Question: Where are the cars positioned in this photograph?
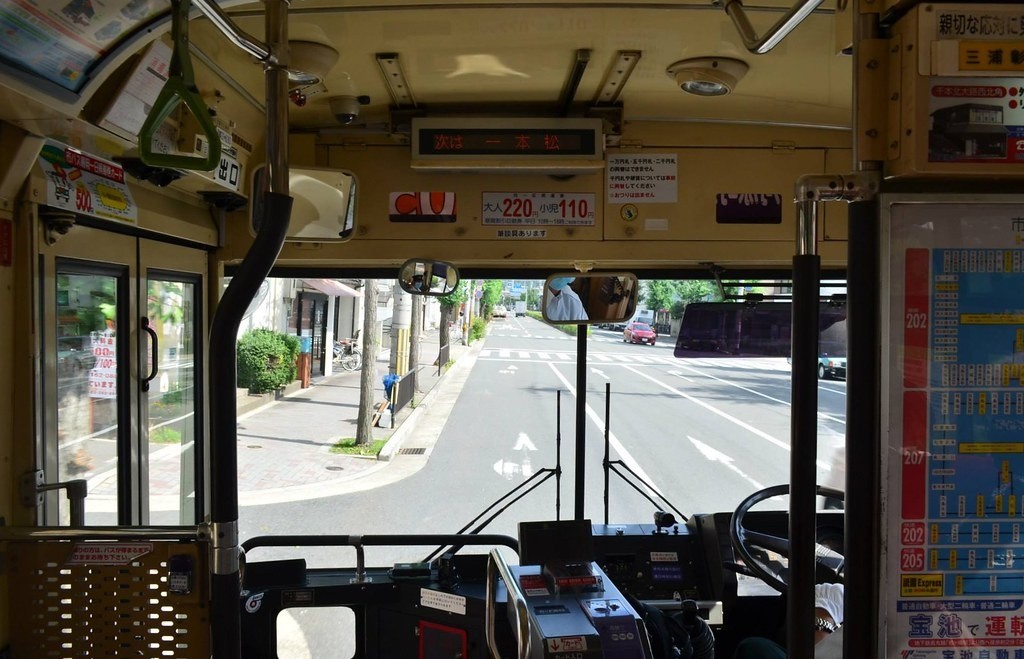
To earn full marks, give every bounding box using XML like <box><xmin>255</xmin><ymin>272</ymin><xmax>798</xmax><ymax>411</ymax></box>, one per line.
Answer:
<box><xmin>56</xmin><ymin>335</ymin><xmax>96</xmax><ymax>377</ymax></box>
<box><xmin>623</xmin><ymin>322</ymin><xmax>656</xmax><ymax>345</ymax></box>
<box><xmin>492</xmin><ymin>306</ymin><xmax>507</xmax><ymax>317</ymax></box>
<box><xmin>787</xmin><ymin>356</ymin><xmax>846</xmax><ymax>379</ymax></box>
<box><xmin>593</xmin><ymin>321</ymin><xmax>627</xmax><ymax>332</ymax></box>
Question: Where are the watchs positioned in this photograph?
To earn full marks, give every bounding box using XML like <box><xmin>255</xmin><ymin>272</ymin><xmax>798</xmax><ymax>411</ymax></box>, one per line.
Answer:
<box><xmin>814</xmin><ymin>617</ymin><xmax>834</xmax><ymax>633</ymax></box>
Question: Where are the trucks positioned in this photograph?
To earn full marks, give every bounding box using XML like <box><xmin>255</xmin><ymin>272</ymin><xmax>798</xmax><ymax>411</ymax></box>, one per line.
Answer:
<box><xmin>516</xmin><ymin>300</ymin><xmax>527</xmax><ymax>318</ymax></box>
<box><xmin>626</xmin><ymin>304</ymin><xmax>655</xmax><ymax>333</ymax></box>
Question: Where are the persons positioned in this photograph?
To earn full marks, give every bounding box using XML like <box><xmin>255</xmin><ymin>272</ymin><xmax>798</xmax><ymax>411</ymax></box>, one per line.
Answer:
<box><xmin>409</xmin><ymin>275</ymin><xmax>423</xmax><ymax>292</ymax></box>
<box><xmin>738</xmin><ymin>578</ymin><xmax>845</xmax><ymax>659</ymax></box>
<box><xmin>545</xmin><ymin>277</ymin><xmax>590</xmax><ymax>320</ymax></box>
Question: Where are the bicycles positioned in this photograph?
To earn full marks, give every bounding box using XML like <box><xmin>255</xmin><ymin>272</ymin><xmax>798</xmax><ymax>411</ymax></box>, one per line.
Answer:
<box><xmin>333</xmin><ymin>338</ymin><xmax>363</xmax><ymax>372</ymax></box>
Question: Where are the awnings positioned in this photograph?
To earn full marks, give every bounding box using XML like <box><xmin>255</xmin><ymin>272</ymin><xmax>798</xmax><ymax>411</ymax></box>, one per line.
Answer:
<box><xmin>300</xmin><ymin>278</ymin><xmax>362</xmax><ymax>298</ymax></box>
